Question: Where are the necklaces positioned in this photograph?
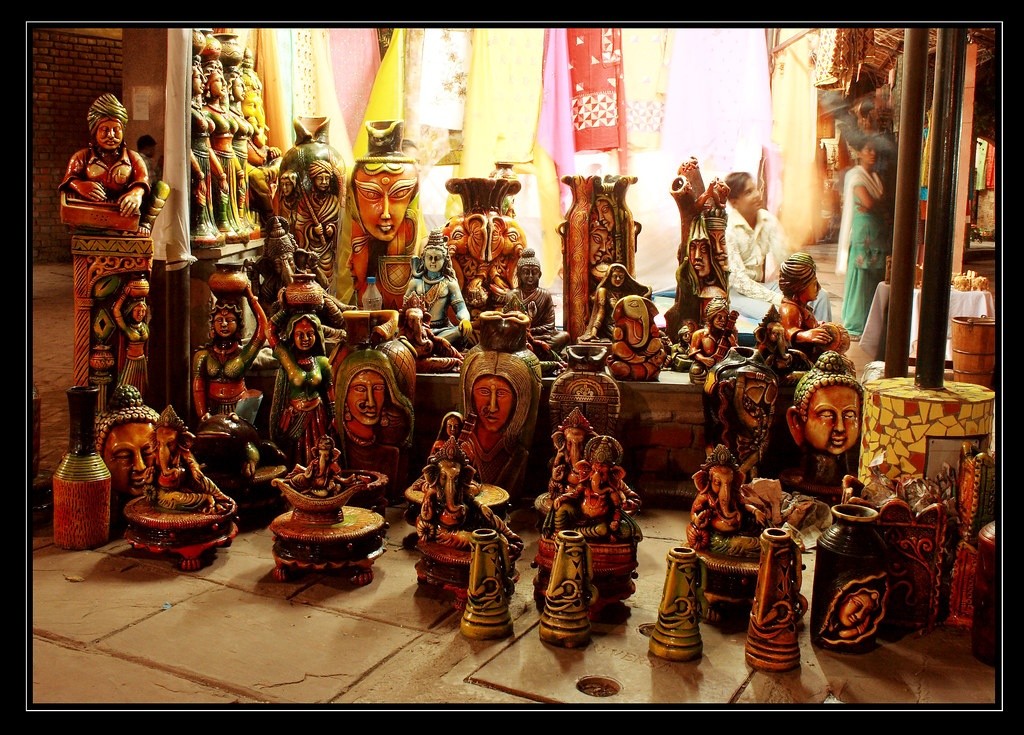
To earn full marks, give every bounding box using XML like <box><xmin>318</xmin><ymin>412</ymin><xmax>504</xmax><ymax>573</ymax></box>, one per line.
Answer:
<box><xmin>422</xmin><ymin>281</ymin><xmax>441</xmax><ymax>312</ymax></box>
<box><xmin>283</xmin><ymin>196</ymin><xmax>296</xmax><ymax>206</ymax></box>
<box><xmin>791</xmin><ymin>297</ymin><xmax>813</xmax><ymax>311</ymax></box>
<box><xmin>296</xmin><ymin>354</ymin><xmax>313</xmax><ymax>365</ymax></box>
<box><xmin>214</xmin><ymin>338</ymin><xmax>238</xmax><ymax>354</ymax></box>
<box><xmin>704</xmin><ymin>278</ymin><xmax>717</xmax><ymax>285</ymax></box>
<box><xmin>209</xmin><ymin>103</ymin><xmax>226</xmax><ymax>115</ymax></box>
<box><xmin>191</xmin><ymin>103</ymin><xmax>201</xmax><ymax>110</ymax></box>
<box><xmin>518</xmin><ymin>288</ymin><xmax>538</xmax><ymax>307</ymax></box>
<box><xmin>229</xmin><ymin>106</ymin><xmax>242</xmax><ymax>116</ymax></box>
<box><xmin>343</xmin><ymin>422</ymin><xmax>376</xmax><ymax>447</ymax></box>
<box><xmin>315</xmin><ymin>194</ymin><xmax>326</xmax><ymax>205</ymax></box>
<box><xmin>130</xmin><ymin>322</ymin><xmax>142</xmax><ymax>327</ymax></box>
<box><xmin>608</xmin><ymin>290</ymin><xmax>621</xmax><ymax>300</ymax></box>
<box><xmin>96</xmin><ymin>148</ymin><xmax>120</xmax><ymax>158</ymax></box>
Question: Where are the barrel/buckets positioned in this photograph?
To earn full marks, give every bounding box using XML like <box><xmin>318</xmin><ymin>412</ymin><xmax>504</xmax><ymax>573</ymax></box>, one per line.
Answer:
<box><xmin>951</xmin><ymin>315</ymin><xmax>995</xmax><ymax>391</ymax></box>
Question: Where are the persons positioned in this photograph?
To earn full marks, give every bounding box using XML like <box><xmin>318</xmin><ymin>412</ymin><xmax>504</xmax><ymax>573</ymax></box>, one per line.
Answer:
<box><xmin>726</xmin><ymin>173</ymin><xmax>831</xmax><ymax>324</ymax></box>
<box><xmin>60</xmin><ymin>66</ymin><xmax>891</xmax><ymax>637</ymax></box>
<box><xmin>135</xmin><ymin>134</ymin><xmax>157</xmax><ymax>186</ymax></box>
<box><xmin>835</xmin><ymin>137</ymin><xmax>887</xmax><ymax>341</ymax></box>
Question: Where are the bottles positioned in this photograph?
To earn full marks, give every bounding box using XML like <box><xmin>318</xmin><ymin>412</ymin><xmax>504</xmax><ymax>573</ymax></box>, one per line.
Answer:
<box><xmin>362</xmin><ymin>277</ymin><xmax>382</xmax><ymax>310</ymax></box>
